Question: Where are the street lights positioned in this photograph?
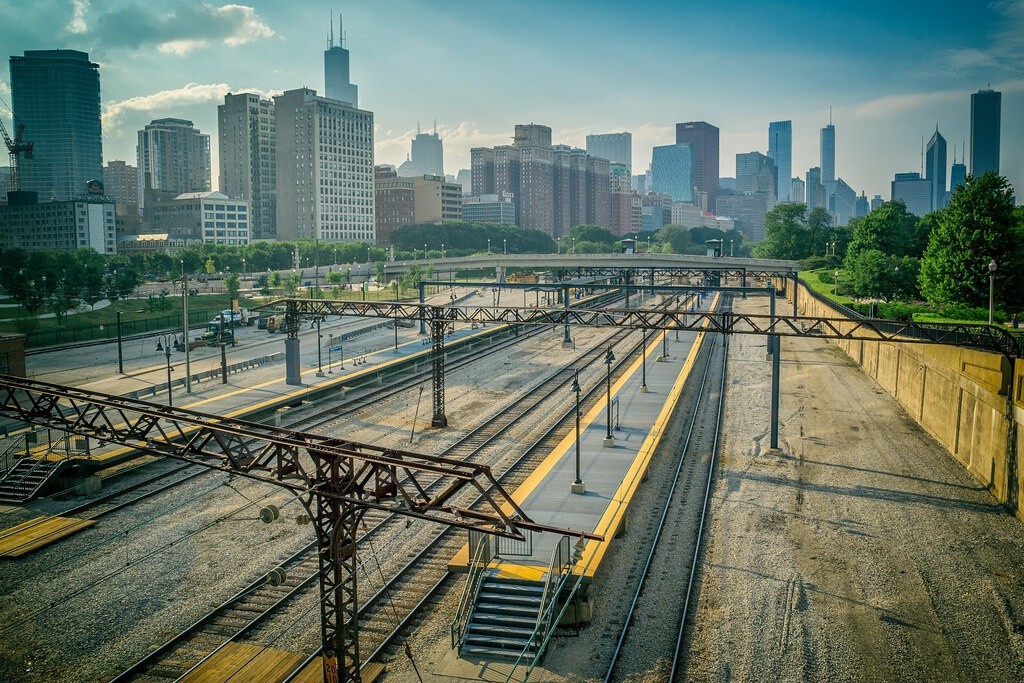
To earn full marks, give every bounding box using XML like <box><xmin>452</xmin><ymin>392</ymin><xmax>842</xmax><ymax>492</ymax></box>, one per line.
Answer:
<box><xmin>557</xmin><ymin>237</ymin><xmax>561</xmax><ymax>253</ymax></box>
<box><xmin>730</xmin><ymin>239</ymin><xmax>734</xmax><ymax>257</ymax></box>
<box><xmin>116</xmin><ymin>309</ymin><xmax>145</xmax><ymax>373</ymax></box>
<box><xmin>647</xmin><ymin>236</ymin><xmax>650</xmax><ymax>253</ymax></box>
<box><xmin>503</xmin><ymin>239</ymin><xmax>507</xmax><ymax>254</ymax></box>
<box><xmin>835</xmin><ymin>271</ymin><xmax>838</xmax><ymax>296</ymax></box>
<box><xmin>291</xmin><ymin>249</ymin><xmax>294</xmax><ymax>269</ymax></box>
<box><xmin>572</xmin><ymin>236</ymin><xmax>575</xmax><ymax>254</ymax></box>
<box><xmin>720</xmin><ymin>238</ymin><xmax>724</xmax><ymax>257</ymax></box>
<box><xmin>635</xmin><ymin>236</ymin><xmax>637</xmax><ymax>253</ymax></box>
<box><xmin>674</xmin><ymin>295</ymin><xmax>680</xmax><ymax>342</ymax></box>
<box><xmin>832</xmin><ymin>242</ymin><xmax>835</xmax><ymax>255</ymax></box>
<box><xmin>311</xmin><ymin>313</ymin><xmax>327</xmax><ymax>377</ymax></box>
<box><xmin>181</xmin><ymin>260</ymin><xmax>184</xmax><ymax>279</ymax></box>
<box><xmin>570</xmin><ymin>366</ymin><xmax>584</xmax><ymax>494</ymax></box>
<box><xmin>641</xmin><ymin>329</ymin><xmax>648</xmax><ymax>393</ymax></box>
<box><xmin>155</xmin><ymin>333</ymin><xmax>181</xmax><ymax>427</ymax></box>
<box><xmin>826</xmin><ymin>242</ymin><xmax>829</xmax><ymax>255</ymax></box>
<box><xmin>602</xmin><ymin>343</ymin><xmax>616</xmax><ymax>447</ymax></box>
<box><xmin>488</xmin><ymin>239</ymin><xmax>491</xmax><ymax>256</ymax></box>
<box><xmin>367</xmin><ymin>243</ymin><xmax>444</xmax><ymax>283</ymax></box>
<box><xmin>333</xmin><ymin>248</ymin><xmax>338</xmax><ymax>271</ymax></box>
<box><xmin>43</xmin><ymin>276</ymin><xmax>48</xmax><ymax>314</ymax></box>
<box><xmin>989</xmin><ymin>259</ymin><xmax>997</xmax><ymax>325</ymax></box>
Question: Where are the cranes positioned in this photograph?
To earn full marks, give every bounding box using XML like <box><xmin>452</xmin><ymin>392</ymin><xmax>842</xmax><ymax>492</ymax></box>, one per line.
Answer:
<box><xmin>0</xmin><ymin>115</ymin><xmax>35</xmax><ymax>193</ymax></box>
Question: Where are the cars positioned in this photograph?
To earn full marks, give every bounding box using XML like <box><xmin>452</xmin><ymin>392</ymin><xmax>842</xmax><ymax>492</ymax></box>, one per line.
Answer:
<box><xmin>179</xmin><ymin>304</ymin><xmax>328</xmax><ymax>352</ymax></box>
